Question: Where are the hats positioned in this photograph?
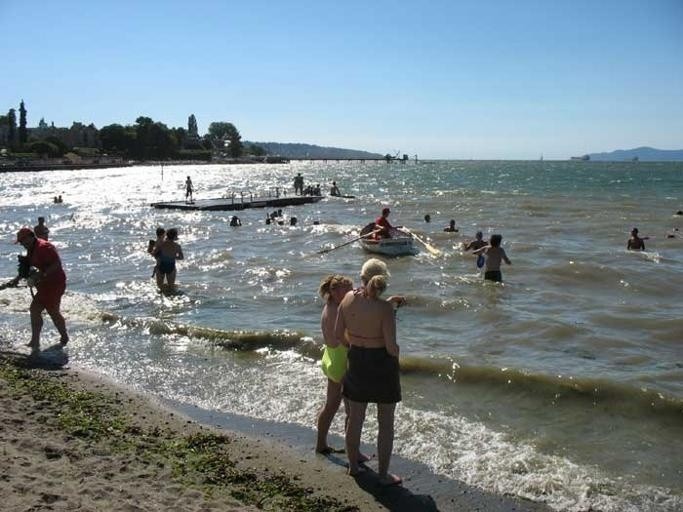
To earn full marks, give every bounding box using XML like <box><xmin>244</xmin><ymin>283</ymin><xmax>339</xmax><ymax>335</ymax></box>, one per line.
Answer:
<box><xmin>383</xmin><ymin>208</ymin><xmax>390</xmax><ymax>213</ymax></box>
<box><xmin>14</xmin><ymin>228</ymin><xmax>31</xmax><ymax>244</ymax></box>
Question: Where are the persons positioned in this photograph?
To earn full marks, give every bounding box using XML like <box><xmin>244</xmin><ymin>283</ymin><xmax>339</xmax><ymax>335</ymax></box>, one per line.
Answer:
<box><xmin>443</xmin><ymin>219</ymin><xmax>458</xmax><ymax>233</ymax></box>
<box><xmin>627</xmin><ymin>227</ymin><xmax>646</xmax><ymax>252</ymax></box>
<box><xmin>53</xmin><ymin>196</ymin><xmax>59</xmax><ymax>203</ymax></box>
<box><xmin>313</xmin><ymin>275</ymin><xmax>408</xmax><ymax>463</ymax></box>
<box><xmin>152</xmin><ymin>228</ymin><xmax>184</xmax><ymax>291</ymax></box>
<box><xmin>463</xmin><ymin>229</ymin><xmax>488</xmax><ymax>251</ymax></box>
<box><xmin>183</xmin><ymin>176</ymin><xmax>196</xmax><ymax>204</ymax></box>
<box><xmin>0</xmin><ymin>226</ymin><xmax>70</xmax><ymax>349</ymax></box>
<box><xmin>373</xmin><ymin>207</ymin><xmax>397</xmax><ymax>238</ymax></box>
<box><xmin>472</xmin><ymin>233</ymin><xmax>511</xmax><ymax>284</ymax></box>
<box><xmin>148</xmin><ymin>227</ymin><xmax>166</xmax><ymax>278</ymax></box>
<box><xmin>263</xmin><ymin>171</ymin><xmax>344</xmax><ymax>227</ymax></box>
<box><xmin>229</xmin><ymin>216</ymin><xmax>242</xmax><ymax>226</ymax></box>
<box><xmin>33</xmin><ymin>215</ymin><xmax>50</xmax><ymax>240</ymax></box>
<box><xmin>58</xmin><ymin>194</ymin><xmax>63</xmax><ymax>202</ymax></box>
<box><xmin>423</xmin><ymin>213</ymin><xmax>431</xmax><ymax>222</ymax></box>
<box><xmin>333</xmin><ymin>256</ymin><xmax>405</xmax><ymax>487</ymax></box>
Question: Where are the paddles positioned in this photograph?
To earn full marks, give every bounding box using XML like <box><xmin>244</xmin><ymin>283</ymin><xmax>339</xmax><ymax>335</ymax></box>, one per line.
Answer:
<box><xmin>316</xmin><ymin>226</ymin><xmax>394</xmax><ymax>257</ymax></box>
<box><xmin>401</xmin><ymin>224</ymin><xmax>443</xmax><ymax>257</ymax></box>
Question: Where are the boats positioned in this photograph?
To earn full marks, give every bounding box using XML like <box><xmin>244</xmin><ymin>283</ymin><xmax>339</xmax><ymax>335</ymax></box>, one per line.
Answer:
<box><xmin>356</xmin><ymin>224</ymin><xmax>417</xmax><ymax>258</ymax></box>
